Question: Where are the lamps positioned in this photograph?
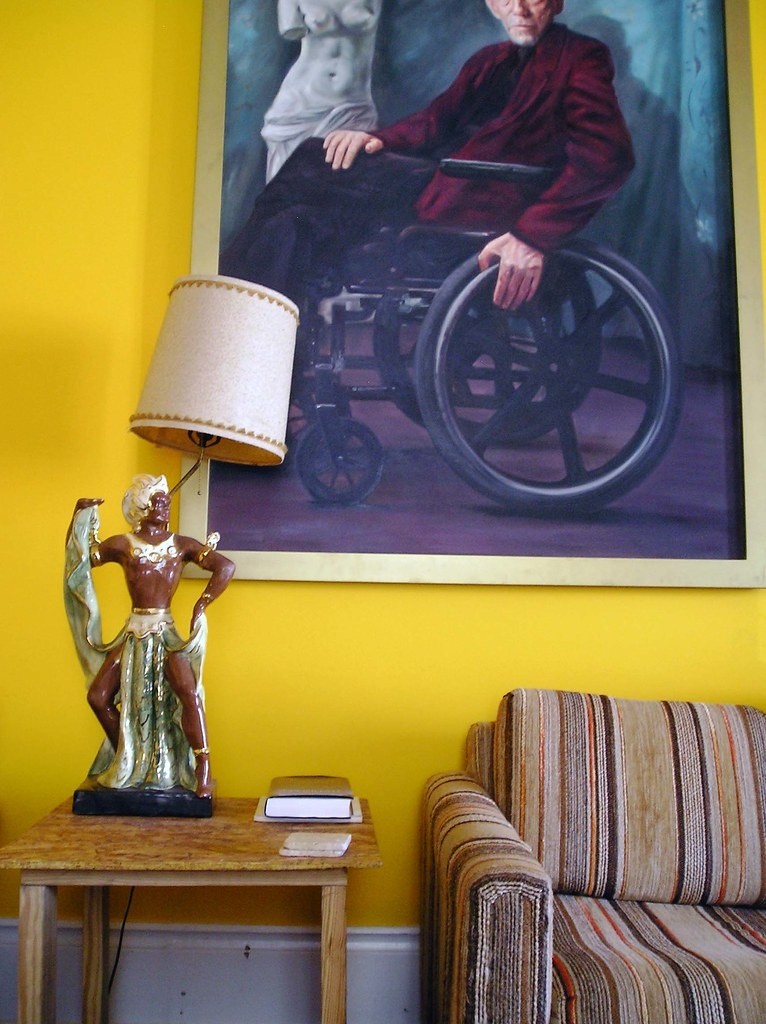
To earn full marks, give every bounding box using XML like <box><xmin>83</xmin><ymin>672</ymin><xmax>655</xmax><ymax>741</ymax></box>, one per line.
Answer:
<box><xmin>59</xmin><ymin>271</ymin><xmax>301</xmax><ymax>808</ymax></box>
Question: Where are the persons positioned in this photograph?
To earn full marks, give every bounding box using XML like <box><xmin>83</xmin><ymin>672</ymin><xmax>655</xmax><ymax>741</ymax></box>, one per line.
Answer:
<box><xmin>65</xmin><ymin>475</ymin><xmax>237</xmax><ymax>800</ymax></box>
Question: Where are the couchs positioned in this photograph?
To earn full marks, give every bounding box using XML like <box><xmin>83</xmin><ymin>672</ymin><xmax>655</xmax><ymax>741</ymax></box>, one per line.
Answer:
<box><xmin>415</xmin><ymin>689</ymin><xmax>766</xmax><ymax>1024</ymax></box>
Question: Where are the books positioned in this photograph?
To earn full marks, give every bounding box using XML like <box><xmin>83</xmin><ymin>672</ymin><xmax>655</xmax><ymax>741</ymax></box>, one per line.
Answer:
<box><xmin>264</xmin><ymin>775</ymin><xmax>354</xmax><ymax>820</ymax></box>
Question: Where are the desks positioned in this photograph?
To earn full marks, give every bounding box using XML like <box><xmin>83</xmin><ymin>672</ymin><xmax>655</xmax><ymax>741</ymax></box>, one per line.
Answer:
<box><xmin>0</xmin><ymin>800</ymin><xmax>381</xmax><ymax>1024</ymax></box>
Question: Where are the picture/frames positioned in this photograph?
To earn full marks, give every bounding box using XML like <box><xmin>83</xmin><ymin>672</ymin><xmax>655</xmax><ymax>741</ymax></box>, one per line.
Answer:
<box><xmin>179</xmin><ymin>1</ymin><xmax>766</xmax><ymax>592</ymax></box>
